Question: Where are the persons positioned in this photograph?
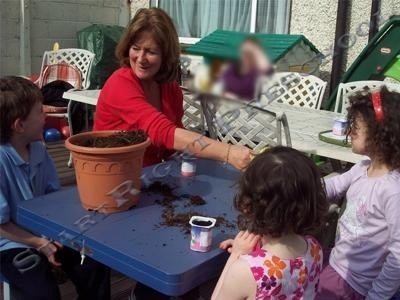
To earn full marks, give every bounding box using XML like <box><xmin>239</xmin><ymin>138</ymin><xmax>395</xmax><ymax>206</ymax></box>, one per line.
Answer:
<box><xmin>210</xmin><ymin>145</ymin><xmax>323</xmax><ymax>300</ymax></box>
<box><xmin>90</xmin><ymin>7</ymin><xmax>254</xmax><ymax>172</ymax></box>
<box><xmin>0</xmin><ymin>74</ymin><xmax>111</xmax><ymax>300</ymax></box>
<box><xmin>321</xmin><ymin>89</ymin><xmax>400</xmax><ymax>300</ymax></box>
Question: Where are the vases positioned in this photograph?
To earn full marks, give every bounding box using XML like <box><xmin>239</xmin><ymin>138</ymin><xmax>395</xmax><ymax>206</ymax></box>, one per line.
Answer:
<box><xmin>61</xmin><ymin>130</ymin><xmax>150</xmax><ymax>213</ymax></box>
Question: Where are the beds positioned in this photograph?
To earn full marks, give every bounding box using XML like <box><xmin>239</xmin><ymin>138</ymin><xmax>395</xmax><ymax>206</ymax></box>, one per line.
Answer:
<box><xmin>63</xmin><ymin>89</ymin><xmax>371</xmax><ymax>166</ymax></box>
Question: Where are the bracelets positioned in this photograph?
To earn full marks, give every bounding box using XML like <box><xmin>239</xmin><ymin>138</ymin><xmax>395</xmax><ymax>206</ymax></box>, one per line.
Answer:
<box><xmin>224</xmin><ymin>143</ymin><xmax>231</xmax><ymax>165</ymax></box>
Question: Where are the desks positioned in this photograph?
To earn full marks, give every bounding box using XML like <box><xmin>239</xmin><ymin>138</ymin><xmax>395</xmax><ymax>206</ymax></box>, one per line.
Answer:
<box><xmin>16</xmin><ymin>157</ymin><xmax>242</xmax><ymax>297</ymax></box>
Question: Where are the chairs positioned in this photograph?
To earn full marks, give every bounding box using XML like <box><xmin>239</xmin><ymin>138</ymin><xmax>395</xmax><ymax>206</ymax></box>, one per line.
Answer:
<box><xmin>34</xmin><ymin>49</ymin><xmax>96</xmax><ymax>166</ymax></box>
<box><xmin>259</xmin><ymin>72</ymin><xmax>328</xmax><ymax>109</ymax></box>
<box><xmin>200</xmin><ymin>94</ymin><xmax>292</xmax><ymax>153</ymax></box>
<box><xmin>335</xmin><ymin>80</ymin><xmax>400</xmax><ymax>114</ymax></box>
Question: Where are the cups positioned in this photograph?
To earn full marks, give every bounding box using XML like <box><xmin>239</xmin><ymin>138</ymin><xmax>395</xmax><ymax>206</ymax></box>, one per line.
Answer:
<box><xmin>189</xmin><ymin>215</ymin><xmax>216</xmax><ymax>252</ymax></box>
<box><xmin>179</xmin><ymin>156</ymin><xmax>198</xmax><ymax>178</ymax></box>
<box><xmin>334</xmin><ymin>119</ymin><xmax>348</xmax><ymax>135</ymax></box>
